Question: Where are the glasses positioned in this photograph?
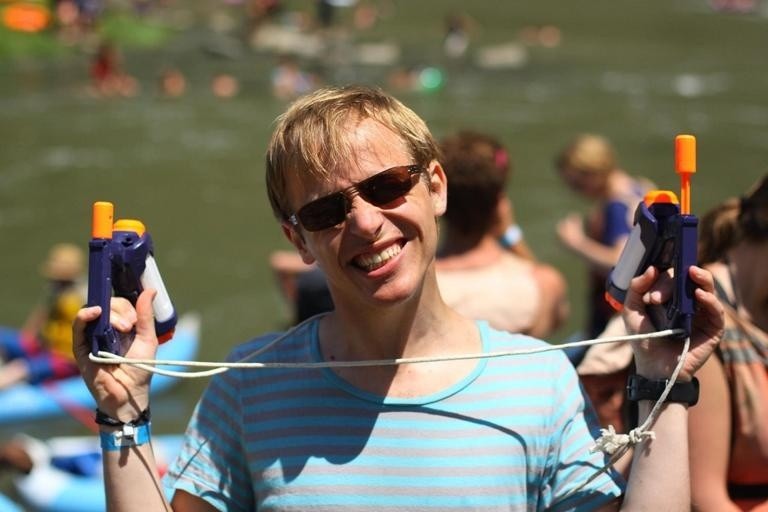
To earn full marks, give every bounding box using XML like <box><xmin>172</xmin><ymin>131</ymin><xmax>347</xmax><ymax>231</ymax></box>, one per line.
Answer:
<box><xmin>285</xmin><ymin>162</ymin><xmax>430</xmax><ymax>234</ymax></box>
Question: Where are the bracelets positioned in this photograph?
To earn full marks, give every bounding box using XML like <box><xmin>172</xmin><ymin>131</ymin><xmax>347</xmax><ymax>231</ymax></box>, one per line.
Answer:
<box><xmin>97</xmin><ymin>422</ymin><xmax>153</xmax><ymax>451</ymax></box>
<box><xmin>92</xmin><ymin>408</ymin><xmax>152</xmax><ymax>427</ymax></box>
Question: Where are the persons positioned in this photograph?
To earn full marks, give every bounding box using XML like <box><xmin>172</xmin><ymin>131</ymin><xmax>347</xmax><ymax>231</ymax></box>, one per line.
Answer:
<box><xmin>428</xmin><ymin>136</ymin><xmax>569</xmax><ymax>345</ymax></box>
<box><xmin>574</xmin><ymin>363</ymin><xmax>631</xmax><ymax>433</ymax></box>
<box><xmin>688</xmin><ymin>173</ymin><xmax>767</xmax><ymax>512</ymax></box>
<box><xmin>70</xmin><ymin>79</ymin><xmax>729</xmax><ymax>510</ymax></box>
<box><xmin>0</xmin><ymin>240</ymin><xmax>86</xmax><ymax>388</ymax></box>
<box><xmin>553</xmin><ymin>132</ymin><xmax>644</xmax><ymax>340</ymax></box>
<box><xmin>270</xmin><ymin>250</ymin><xmax>333</xmax><ymax>323</ymax></box>
